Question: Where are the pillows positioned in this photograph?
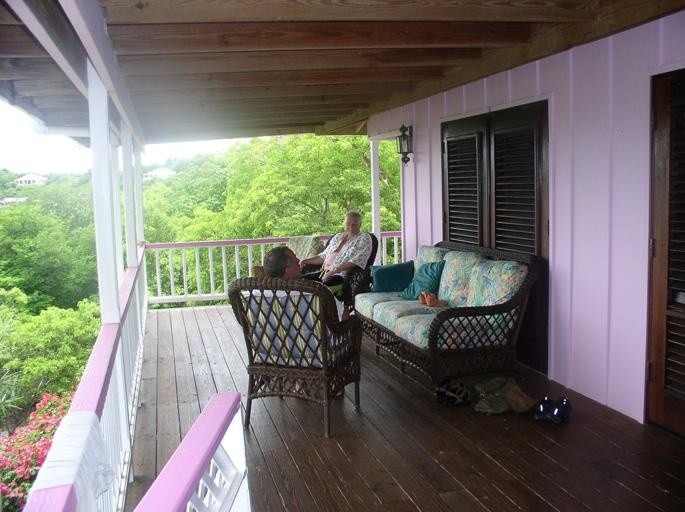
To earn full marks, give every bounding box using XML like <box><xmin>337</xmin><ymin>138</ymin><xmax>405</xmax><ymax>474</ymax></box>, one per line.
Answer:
<box><xmin>369</xmin><ymin>260</ymin><xmax>446</xmax><ymax>300</ymax></box>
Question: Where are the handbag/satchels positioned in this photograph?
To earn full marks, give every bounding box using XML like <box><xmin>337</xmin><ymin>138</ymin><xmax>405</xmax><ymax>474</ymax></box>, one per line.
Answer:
<box><xmin>474</xmin><ymin>377</ymin><xmax>534</xmax><ymax>414</ymax></box>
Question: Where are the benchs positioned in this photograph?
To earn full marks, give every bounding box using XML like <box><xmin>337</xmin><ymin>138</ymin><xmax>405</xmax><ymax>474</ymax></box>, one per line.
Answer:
<box><xmin>355</xmin><ymin>241</ymin><xmax>543</xmax><ymax>404</ymax></box>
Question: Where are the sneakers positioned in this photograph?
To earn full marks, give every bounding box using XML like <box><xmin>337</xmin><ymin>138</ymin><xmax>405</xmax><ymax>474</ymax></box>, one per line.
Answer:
<box><xmin>534</xmin><ymin>393</ymin><xmax>570</xmax><ymax>424</ymax></box>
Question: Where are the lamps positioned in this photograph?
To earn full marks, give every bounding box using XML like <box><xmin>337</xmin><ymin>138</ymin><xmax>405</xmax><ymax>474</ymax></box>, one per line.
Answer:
<box><xmin>396</xmin><ymin>123</ymin><xmax>413</xmax><ymax>165</ymax></box>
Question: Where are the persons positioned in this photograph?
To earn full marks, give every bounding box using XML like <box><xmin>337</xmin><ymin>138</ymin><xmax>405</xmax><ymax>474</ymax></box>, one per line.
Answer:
<box><xmin>300</xmin><ymin>210</ymin><xmax>373</xmax><ymax>287</ymax></box>
<box><xmin>263</xmin><ymin>246</ymin><xmax>351</xmax><ymax>398</ymax></box>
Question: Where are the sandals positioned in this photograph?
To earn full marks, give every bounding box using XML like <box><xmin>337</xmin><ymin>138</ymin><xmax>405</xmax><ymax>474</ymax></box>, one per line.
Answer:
<box><xmin>435</xmin><ymin>380</ymin><xmax>471</xmax><ymax>408</ymax></box>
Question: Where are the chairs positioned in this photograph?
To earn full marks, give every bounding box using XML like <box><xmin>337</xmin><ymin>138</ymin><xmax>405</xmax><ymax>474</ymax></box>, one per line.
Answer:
<box><xmin>227</xmin><ymin>275</ymin><xmax>363</xmax><ymax>439</ymax></box>
<box><xmin>301</xmin><ymin>232</ymin><xmax>378</xmax><ymax>314</ymax></box>
